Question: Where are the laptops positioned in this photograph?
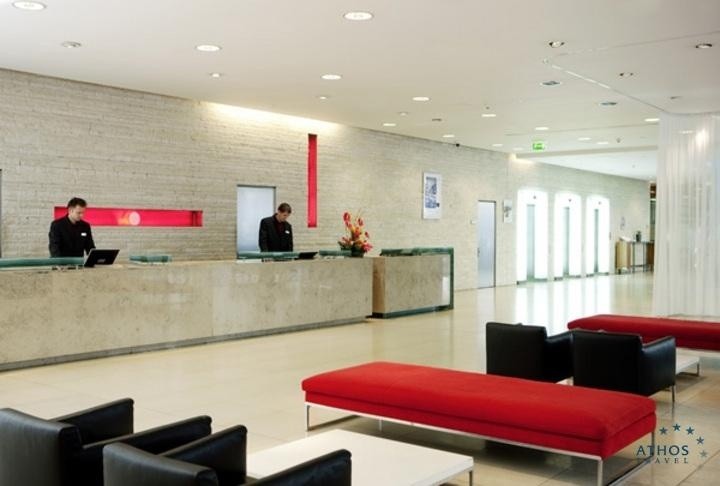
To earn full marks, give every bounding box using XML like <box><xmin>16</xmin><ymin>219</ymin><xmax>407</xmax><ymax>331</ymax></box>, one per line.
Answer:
<box><xmin>84</xmin><ymin>250</ymin><xmax>119</xmax><ymax>267</ymax></box>
<box><xmin>295</xmin><ymin>253</ymin><xmax>317</xmax><ymax>259</ymax></box>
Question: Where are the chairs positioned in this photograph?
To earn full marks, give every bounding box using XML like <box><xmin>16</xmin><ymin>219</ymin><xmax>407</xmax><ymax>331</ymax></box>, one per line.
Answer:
<box><xmin>0</xmin><ymin>395</ymin><xmax>352</xmax><ymax>486</ymax></box>
<box><xmin>485</xmin><ymin>319</ymin><xmax>678</xmax><ymax>415</ymax></box>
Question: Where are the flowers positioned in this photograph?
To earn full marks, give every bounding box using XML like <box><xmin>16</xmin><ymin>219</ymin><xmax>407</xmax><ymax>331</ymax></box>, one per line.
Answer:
<box><xmin>337</xmin><ymin>209</ymin><xmax>374</xmax><ymax>253</ymax></box>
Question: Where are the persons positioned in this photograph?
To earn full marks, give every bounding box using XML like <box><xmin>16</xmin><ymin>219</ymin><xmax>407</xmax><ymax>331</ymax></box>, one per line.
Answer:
<box><xmin>48</xmin><ymin>197</ymin><xmax>97</xmax><ymax>258</ymax></box>
<box><xmin>258</xmin><ymin>203</ymin><xmax>294</xmax><ymax>252</ymax></box>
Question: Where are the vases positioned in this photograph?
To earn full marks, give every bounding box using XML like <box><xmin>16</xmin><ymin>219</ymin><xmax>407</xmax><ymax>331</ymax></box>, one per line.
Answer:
<box><xmin>351</xmin><ymin>249</ymin><xmax>364</xmax><ymax>257</ymax></box>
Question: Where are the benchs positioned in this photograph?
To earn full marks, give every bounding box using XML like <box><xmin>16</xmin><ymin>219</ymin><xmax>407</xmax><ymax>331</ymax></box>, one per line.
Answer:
<box><xmin>302</xmin><ymin>361</ymin><xmax>659</xmax><ymax>486</ymax></box>
<box><xmin>567</xmin><ymin>313</ymin><xmax>720</xmax><ymax>355</ymax></box>
